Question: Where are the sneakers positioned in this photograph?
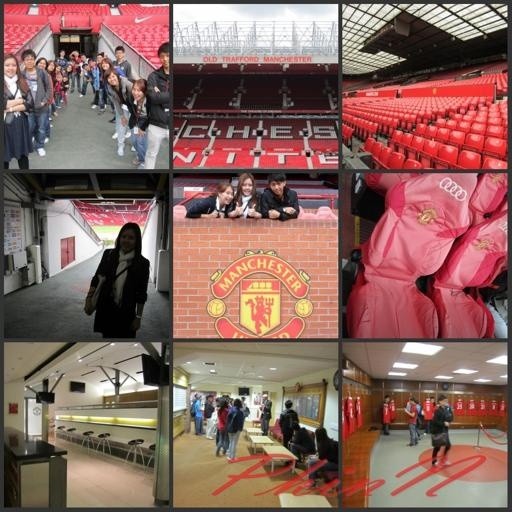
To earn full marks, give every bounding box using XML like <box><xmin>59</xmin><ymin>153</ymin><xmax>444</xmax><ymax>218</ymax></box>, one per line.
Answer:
<box><xmin>132</xmin><ymin>156</ymin><xmax>144</xmax><ymax>169</ymax></box>
<box><xmin>37</xmin><ymin>137</ymin><xmax>50</xmax><ymax>156</ymax></box>
<box><xmin>117</xmin><ymin>146</ymin><xmax>124</xmax><ymax>156</ymax></box>
<box><xmin>79</xmin><ymin>93</ymin><xmax>83</xmax><ymax>97</ymax></box>
<box><xmin>131</xmin><ymin>146</ymin><xmax>136</xmax><ymax>151</ymax></box>
<box><xmin>49</xmin><ymin>99</ymin><xmax>64</xmax><ymax>129</ymax></box>
<box><xmin>112</xmin><ymin>132</ymin><xmax>118</xmax><ymax>139</ymax></box>
<box><xmin>91</xmin><ymin>104</ymin><xmax>106</xmax><ymax>112</ymax></box>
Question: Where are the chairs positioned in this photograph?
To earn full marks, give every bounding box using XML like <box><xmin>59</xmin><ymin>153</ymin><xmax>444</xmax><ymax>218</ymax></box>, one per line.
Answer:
<box><xmin>342</xmin><ymin>174</ymin><xmax>508</xmax><ymax>338</ymax></box>
<box><xmin>342</xmin><ymin>63</ymin><xmax>508</xmax><ymax>170</ymax></box>
<box><xmin>4</xmin><ymin>4</ymin><xmax>169</xmax><ymax>75</ymax></box>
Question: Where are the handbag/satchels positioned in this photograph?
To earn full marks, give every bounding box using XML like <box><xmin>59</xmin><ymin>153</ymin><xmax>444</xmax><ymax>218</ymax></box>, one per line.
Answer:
<box><xmin>3</xmin><ymin>112</ymin><xmax>15</xmax><ymax>125</ymax></box>
<box><xmin>91</xmin><ymin>274</ymin><xmax>107</xmax><ymax>309</ymax></box>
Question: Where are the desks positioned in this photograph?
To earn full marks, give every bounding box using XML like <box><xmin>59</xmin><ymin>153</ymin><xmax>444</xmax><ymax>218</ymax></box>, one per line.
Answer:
<box><xmin>246</xmin><ymin>427</ymin><xmax>264</xmax><ymax>441</ymax></box>
<box><xmin>250</xmin><ymin>435</ymin><xmax>274</xmax><ymax>454</ymax></box>
<box><xmin>262</xmin><ymin>445</ymin><xmax>298</xmax><ymax>473</ymax></box>
<box><xmin>279</xmin><ymin>493</ymin><xmax>332</xmax><ymax>508</ymax></box>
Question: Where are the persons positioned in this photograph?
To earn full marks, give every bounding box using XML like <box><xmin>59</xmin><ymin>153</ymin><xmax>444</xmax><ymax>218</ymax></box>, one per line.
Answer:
<box><xmin>84</xmin><ymin>223</ymin><xmax>150</xmax><ymax>339</ymax></box>
<box><xmin>185</xmin><ymin>170</ymin><xmax>300</xmax><ymax>222</ymax></box>
<box><xmin>4</xmin><ymin>42</ymin><xmax>170</xmax><ymax>169</ymax></box>
<box><xmin>382</xmin><ymin>395</ymin><xmax>453</xmax><ymax>466</ymax></box>
<box><xmin>191</xmin><ymin>392</ymin><xmax>339</xmax><ymax>489</ymax></box>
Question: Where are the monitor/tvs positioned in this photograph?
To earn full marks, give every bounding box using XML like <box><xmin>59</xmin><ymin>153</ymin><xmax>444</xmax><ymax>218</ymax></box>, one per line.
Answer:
<box><xmin>36</xmin><ymin>392</ymin><xmax>55</xmax><ymax>403</ymax></box>
<box><xmin>70</xmin><ymin>381</ymin><xmax>86</xmax><ymax>393</ymax></box>
<box><xmin>239</xmin><ymin>388</ymin><xmax>250</xmax><ymax>396</ymax></box>
<box><xmin>141</xmin><ymin>353</ymin><xmax>169</xmax><ymax>386</ymax></box>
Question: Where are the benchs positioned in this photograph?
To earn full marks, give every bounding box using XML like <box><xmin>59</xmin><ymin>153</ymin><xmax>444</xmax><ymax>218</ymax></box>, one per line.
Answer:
<box><xmin>391</xmin><ymin>422</ymin><xmax>498</xmax><ymax>430</ymax></box>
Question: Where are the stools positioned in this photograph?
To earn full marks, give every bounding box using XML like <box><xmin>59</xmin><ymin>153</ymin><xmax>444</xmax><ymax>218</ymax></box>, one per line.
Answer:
<box><xmin>57</xmin><ymin>426</ymin><xmax>155</xmax><ymax>474</ymax></box>
